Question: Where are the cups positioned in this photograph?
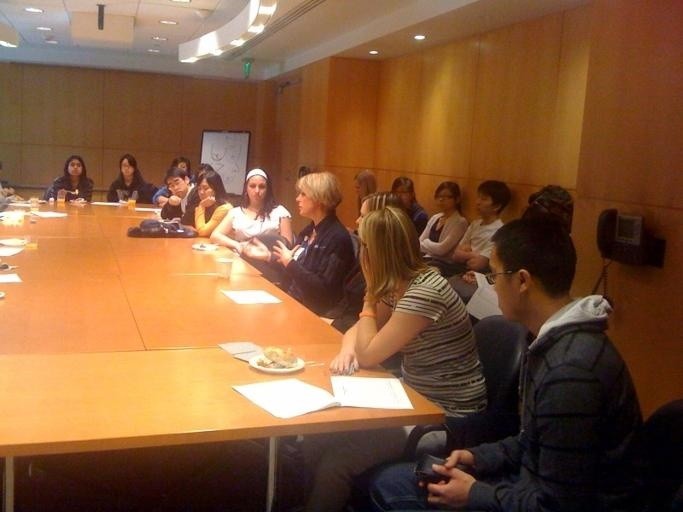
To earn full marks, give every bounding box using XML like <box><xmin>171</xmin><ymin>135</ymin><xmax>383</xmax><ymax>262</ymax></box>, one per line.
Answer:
<box><xmin>56</xmin><ymin>191</ymin><xmax>65</xmax><ymax>202</ymax></box>
<box><xmin>216</xmin><ymin>258</ymin><xmax>234</xmax><ymax>279</ymax></box>
<box><xmin>127</xmin><ymin>196</ymin><xmax>135</xmax><ymax>209</ymax></box>
<box><xmin>30</xmin><ymin>197</ymin><xmax>37</xmax><ymax>211</ymax></box>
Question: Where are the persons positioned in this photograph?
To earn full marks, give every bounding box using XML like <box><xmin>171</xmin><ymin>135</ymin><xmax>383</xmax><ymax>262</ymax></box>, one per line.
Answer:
<box><xmin>43</xmin><ymin>153</ymin><xmax>93</xmax><ymax>204</ymax></box>
<box><xmin>271</xmin><ymin>170</ymin><xmax>355</xmax><ymax>315</ymax></box>
<box><xmin>0</xmin><ymin>161</ymin><xmax>15</xmax><ymax>201</ymax></box>
<box><xmin>353</xmin><ymin>212</ymin><xmax>644</xmax><ymax>511</ymax></box>
<box><xmin>106</xmin><ymin>153</ymin><xmax>158</xmax><ymax>205</ymax></box>
<box><xmin>353</xmin><ymin>172</ymin><xmax>511</xmax><ymax>273</ymax></box>
<box><xmin>208</xmin><ymin>169</ymin><xmax>293</xmax><ymax>265</ymax></box>
<box><xmin>449</xmin><ymin>185</ymin><xmax>572</xmax><ymax>301</ymax></box>
<box><xmin>154</xmin><ymin>157</ymin><xmax>234</xmax><ymax>237</ymax></box>
<box><xmin>300</xmin><ymin>204</ymin><xmax>489</xmax><ymax>512</ymax></box>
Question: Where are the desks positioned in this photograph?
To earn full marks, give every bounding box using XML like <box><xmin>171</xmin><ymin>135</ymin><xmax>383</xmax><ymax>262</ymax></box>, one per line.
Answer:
<box><xmin>1</xmin><ymin>196</ymin><xmax>444</xmax><ymax>512</ymax></box>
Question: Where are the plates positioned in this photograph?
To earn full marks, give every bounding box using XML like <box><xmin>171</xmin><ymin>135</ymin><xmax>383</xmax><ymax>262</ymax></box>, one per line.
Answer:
<box><xmin>191</xmin><ymin>243</ymin><xmax>218</xmax><ymax>250</ymax></box>
<box><xmin>0</xmin><ymin>238</ymin><xmax>27</xmax><ymax>246</ymax></box>
<box><xmin>68</xmin><ymin>199</ymin><xmax>86</xmax><ymax>203</ymax></box>
<box><xmin>248</xmin><ymin>351</ymin><xmax>303</xmax><ymax>373</ymax></box>
<box><xmin>5</xmin><ymin>203</ymin><xmax>30</xmax><ymax>207</ymax></box>
<box><xmin>26</xmin><ymin>199</ymin><xmax>45</xmax><ymax>203</ymax></box>
<box><xmin>0</xmin><ymin>210</ymin><xmax>24</xmax><ymax>217</ymax></box>
<box><xmin>0</xmin><ymin>291</ymin><xmax>5</xmax><ymax>298</ymax></box>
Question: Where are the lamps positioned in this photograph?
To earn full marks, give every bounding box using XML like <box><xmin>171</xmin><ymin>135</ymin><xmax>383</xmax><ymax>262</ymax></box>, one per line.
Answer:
<box><xmin>177</xmin><ymin>1</ymin><xmax>278</xmax><ymax>63</ymax></box>
<box><xmin>0</xmin><ymin>22</ymin><xmax>20</xmax><ymax>48</ymax></box>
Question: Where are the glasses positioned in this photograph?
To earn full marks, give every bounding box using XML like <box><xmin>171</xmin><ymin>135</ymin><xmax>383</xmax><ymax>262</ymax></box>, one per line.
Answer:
<box><xmin>484</xmin><ymin>268</ymin><xmax>513</xmax><ymax>284</ymax></box>
<box><xmin>436</xmin><ymin>195</ymin><xmax>453</xmax><ymax>200</ymax></box>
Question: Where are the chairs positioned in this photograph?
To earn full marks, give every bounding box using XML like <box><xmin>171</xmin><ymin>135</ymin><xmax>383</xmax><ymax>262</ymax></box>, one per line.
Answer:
<box><xmin>240</xmin><ymin>231</ymin><xmax>683</xmax><ymax>511</ymax></box>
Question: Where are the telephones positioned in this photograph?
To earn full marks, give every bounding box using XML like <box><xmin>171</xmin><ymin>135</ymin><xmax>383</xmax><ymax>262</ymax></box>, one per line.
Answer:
<box><xmin>596</xmin><ymin>208</ymin><xmax>644</xmax><ymax>264</ymax></box>
